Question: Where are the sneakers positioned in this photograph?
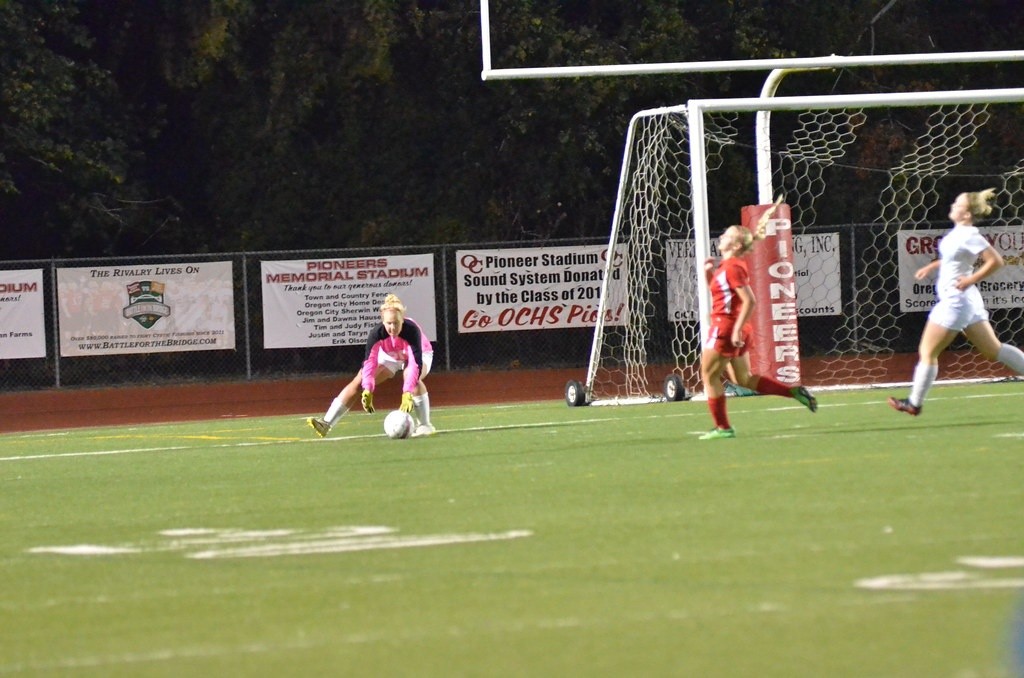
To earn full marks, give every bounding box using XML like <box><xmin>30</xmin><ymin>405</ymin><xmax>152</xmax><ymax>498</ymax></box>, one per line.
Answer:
<box><xmin>307</xmin><ymin>418</ymin><xmax>331</xmax><ymax>438</ymax></box>
<box><xmin>412</xmin><ymin>423</ymin><xmax>435</xmax><ymax>437</ymax></box>
<box><xmin>791</xmin><ymin>386</ymin><xmax>816</xmax><ymax>412</ymax></box>
<box><xmin>888</xmin><ymin>397</ymin><xmax>921</xmax><ymax>416</ymax></box>
<box><xmin>699</xmin><ymin>427</ymin><xmax>735</xmax><ymax>440</ymax></box>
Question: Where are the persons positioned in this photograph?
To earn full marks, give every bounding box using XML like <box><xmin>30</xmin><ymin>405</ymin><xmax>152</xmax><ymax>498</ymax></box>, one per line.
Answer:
<box><xmin>306</xmin><ymin>293</ymin><xmax>435</xmax><ymax>438</ymax></box>
<box><xmin>887</xmin><ymin>187</ymin><xmax>1024</xmax><ymax>416</ymax></box>
<box><xmin>697</xmin><ymin>224</ymin><xmax>819</xmax><ymax>441</ymax></box>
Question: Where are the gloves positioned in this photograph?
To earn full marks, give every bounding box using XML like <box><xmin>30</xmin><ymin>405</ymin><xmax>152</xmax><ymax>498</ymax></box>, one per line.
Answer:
<box><xmin>361</xmin><ymin>390</ymin><xmax>375</xmax><ymax>413</ymax></box>
<box><xmin>400</xmin><ymin>392</ymin><xmax>415</xmax><ymax>412</ymax></box>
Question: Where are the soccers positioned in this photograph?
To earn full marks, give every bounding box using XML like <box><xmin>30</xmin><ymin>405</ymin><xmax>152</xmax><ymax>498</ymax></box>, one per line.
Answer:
<box><xmin>383</xmin><ymin>410</ymin><xmax>414</xmax><ymax>439</ymax></box>
